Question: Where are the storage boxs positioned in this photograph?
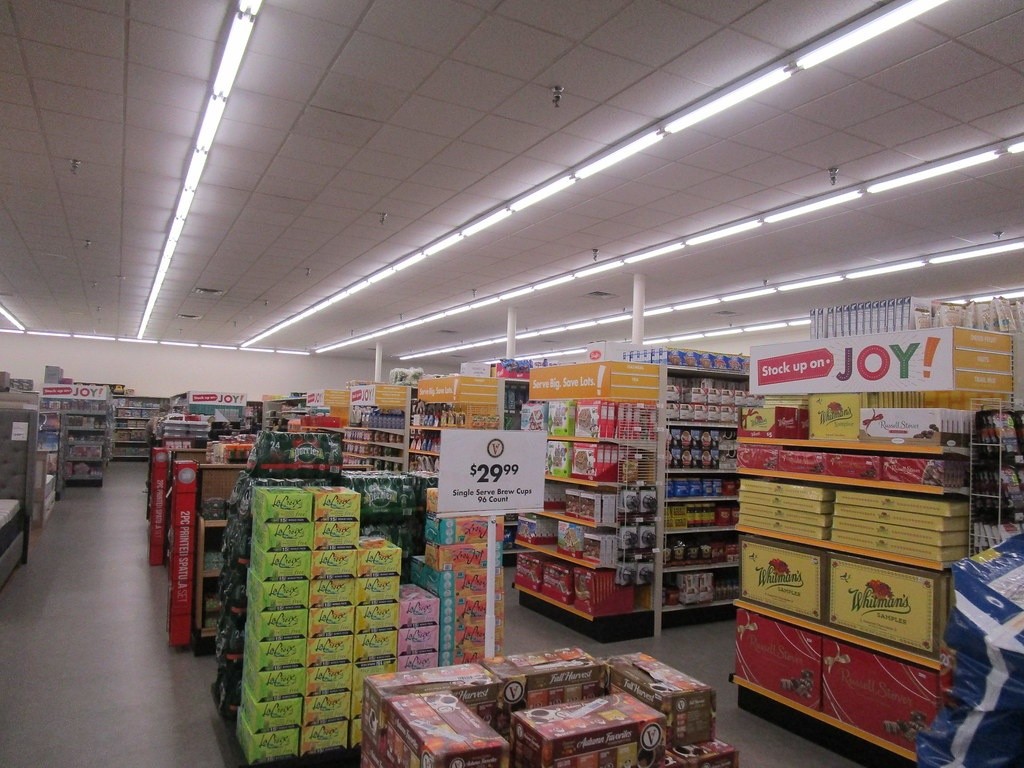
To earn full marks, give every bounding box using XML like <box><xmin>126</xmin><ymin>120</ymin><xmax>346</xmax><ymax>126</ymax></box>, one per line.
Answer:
<box><xmin>236</xmin><ymin>408</ymin><xmax>974</xmax><ymax>768</ymax></box>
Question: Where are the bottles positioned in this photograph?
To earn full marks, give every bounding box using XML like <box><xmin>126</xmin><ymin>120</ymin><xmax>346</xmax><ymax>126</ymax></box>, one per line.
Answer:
<box><xmin>211</xmin><ymin>429</ymin><xmax>439</xmax><ymax>722</ymax></box>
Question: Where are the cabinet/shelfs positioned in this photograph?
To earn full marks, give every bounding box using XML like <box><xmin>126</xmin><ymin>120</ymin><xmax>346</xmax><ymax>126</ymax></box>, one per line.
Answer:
<box><xmin>197</xmin><ymin>510</ymin><xmax>229</xmax><ymax>644</ymax></box>
<box><xmin>62</xmin><ymin>410</ymin><xmax>109</xmax><ymax>487</ymax></box>
<box><xmin>728</xmin><ymin>437</ymin><xmax>968</xmax><ymax>763</ymax></box>
<box><xmin>111</xmin><ymin>395</ymin><xmax>170</xmax><ymax>462</ymax></box>
<box><xmin>497</xmin><ymin>377</ymin><xmax>541</xmax><ymax>568</ymax></box>
<box><xmin>654</xmin><ymin>361</ymin><xmax>751</xmax><ymax>640</ymax></box>
<box><xmin>407</xmin><ymin>425</ymin><xmax>469</xmax><ymax>477</ymax></box>
<box><xmin>341</xmin><ymin>427</ymin><xmax>405</xmax><ymax>471</ymax></box>
<box><xmin>264</xmin><ymin>397</ymin><xmax>308</xmax><ymax>432</ymax></box>
